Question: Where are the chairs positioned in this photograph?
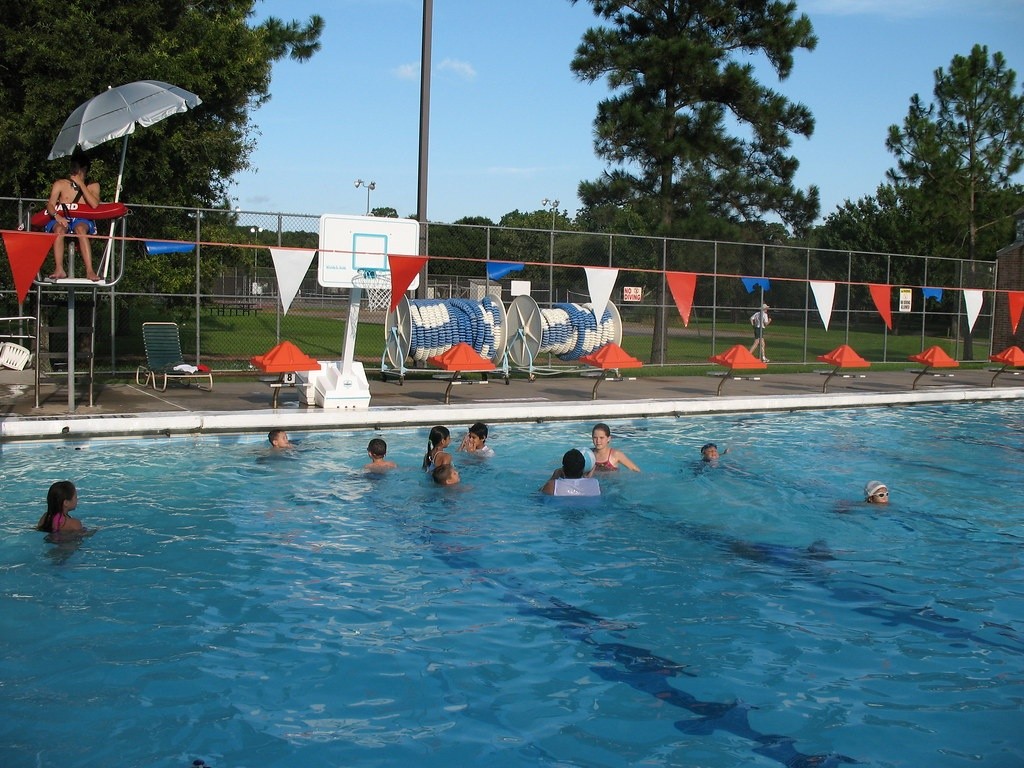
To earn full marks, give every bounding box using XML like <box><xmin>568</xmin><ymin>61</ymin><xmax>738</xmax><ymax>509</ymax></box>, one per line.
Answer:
<box><xmin>136</xmin><ymin>322</ymin><xmax>213</xmax><ymax>391</ymax></box>
<box><xmin>0</xmin><ymin>342</ymin><xmax>35</xmax><ymax>371</ymax></box>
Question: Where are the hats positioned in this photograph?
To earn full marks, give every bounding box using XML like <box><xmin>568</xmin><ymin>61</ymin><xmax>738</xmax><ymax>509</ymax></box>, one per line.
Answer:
<box><xmin>865</xmin><ymin>480</ymin><xmax>887</xmax><ymax>497</ymax></box>
<box><xmin>763</xmin><ymin>304</ymin><xmax>770</xmax><ymax>308</ymax></box>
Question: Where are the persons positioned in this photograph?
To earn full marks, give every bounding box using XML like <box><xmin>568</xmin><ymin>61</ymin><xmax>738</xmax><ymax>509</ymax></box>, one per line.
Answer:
<box><xmin>701</xmin><ymin>443</ymin><xmax>719</xmax><ymax>459</ymax></box>
<box><xmin>589</xmin><ymin>424</ymin><xmax>639</xmax><ymax>470</ymax></box>
<box><xmin>543</xmin><ymin>449</ymin><xmax>602</xmax><ymax>494</ymax></box>
<box><xmin>268</xmin><ymin>430</ymin><xmax>288</xmax><ymax>447</ymax></box>
<box><xmin>47</xmin><ymin>155</ymin><xmax>100</xmax><ymax>280</ymax></box>
<box><xmin>751</xmin><ymin>304</ymin><xmax>772</xmax><ymax>362</ymax></box>
<box><xmin>365</xmin><ymin>439</ymin><xmax>397</xmax><ymax>466</ymax></box>
<box><xmin>421</xmin><ymin>425</ymin><xmax>451</xmax><ymax>468</ymax></box>
<box><xmin>458</xmin><ymin>423</ymin><xmax>493</xmax><ymax>454</ymax></box>
<box><xmin>37</xmin><ymin>481</ymin><xmax>82</xmax><ymax>530</ymax></box>
<box><xmin>864</xmin><ymin>480</ymin><xmax>889</xmax><ymax>502</ymax></box>
<box><xmin>434</xmin><ymin>464</ymin><xmax>459</xmax><ymax>485</ymax></box>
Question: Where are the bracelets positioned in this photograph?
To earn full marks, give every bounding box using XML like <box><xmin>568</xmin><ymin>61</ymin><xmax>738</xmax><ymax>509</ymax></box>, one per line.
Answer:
<box><xmin>52</xmin><ymin>212</ymin><xmax>58</xmax><ymax>217</ymax></box>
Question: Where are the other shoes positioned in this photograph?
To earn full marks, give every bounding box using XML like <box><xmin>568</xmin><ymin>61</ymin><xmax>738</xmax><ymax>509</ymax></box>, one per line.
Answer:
<box><xmin>763</xmin><ymin>360</ymin><xmax>770</xmax><ymax>363</ymax></box>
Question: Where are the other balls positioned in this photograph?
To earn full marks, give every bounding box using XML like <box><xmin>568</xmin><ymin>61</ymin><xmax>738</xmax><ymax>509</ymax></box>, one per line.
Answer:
<box><xmin>575</xmin><ymin>446</ymin><xmax>596</xmax><ymax>473</ymax></box>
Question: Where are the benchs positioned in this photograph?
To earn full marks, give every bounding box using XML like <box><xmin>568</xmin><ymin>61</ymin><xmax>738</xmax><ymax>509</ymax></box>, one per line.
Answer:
<box><xmin>210</xmin><ymin>307</ymin><xmax>262</xmax><ymax>316</ymax></box>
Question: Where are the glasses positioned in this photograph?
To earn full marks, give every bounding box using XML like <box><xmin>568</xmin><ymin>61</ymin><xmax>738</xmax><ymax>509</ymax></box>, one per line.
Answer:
<box><xmin>871</xmin><ymin>492</ymin><xmax>888</xmax><ymax>498</ymax></box>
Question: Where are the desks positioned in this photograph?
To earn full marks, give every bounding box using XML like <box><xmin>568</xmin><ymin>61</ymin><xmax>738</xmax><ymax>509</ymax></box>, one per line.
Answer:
<box><xmin>215</xmin><ymin>300</ymin><xmax>257</xmax><ymax>315</ymax></box>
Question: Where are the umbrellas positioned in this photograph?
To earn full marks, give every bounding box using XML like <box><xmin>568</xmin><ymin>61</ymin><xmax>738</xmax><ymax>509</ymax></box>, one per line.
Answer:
<box><xmin>48</xmin><ymin>80</ymin><xmax>202</xmax><ymax>281</ymax></box>
